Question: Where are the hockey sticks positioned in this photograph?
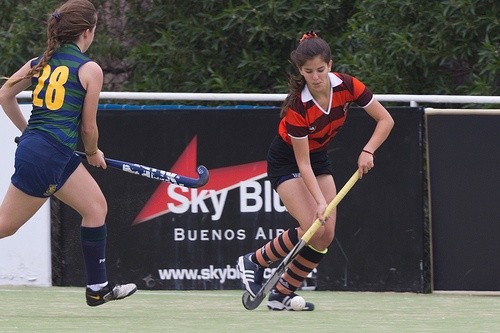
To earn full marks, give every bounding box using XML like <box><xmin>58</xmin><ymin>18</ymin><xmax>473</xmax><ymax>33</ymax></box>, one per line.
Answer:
<box><xmin>14</xmin><ymin>137</ymin><xmax>209</xmax><ymax>187</ymax></box>
<box><xmin>242</xmin><ymin>168</ymin><xmax>360</xmax><ymax>310</ymax></box>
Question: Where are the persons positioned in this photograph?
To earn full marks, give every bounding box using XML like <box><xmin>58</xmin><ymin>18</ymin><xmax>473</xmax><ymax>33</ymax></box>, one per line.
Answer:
<box><xmin>0</xmin><ymin>1</ymin><xmax>137</xmax><ymax>302</ymax></box>
<box><xmin>237</xmin><ymin>32</ymin><xmax>394</xmax><ymax>312</ymax></box>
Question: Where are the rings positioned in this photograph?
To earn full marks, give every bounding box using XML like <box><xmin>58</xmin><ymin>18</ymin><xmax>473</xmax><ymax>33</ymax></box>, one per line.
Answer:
<box><xmin>361</xmin><ymin>150</ymin><xmax>377</xmax><ymax>158</ymax></box>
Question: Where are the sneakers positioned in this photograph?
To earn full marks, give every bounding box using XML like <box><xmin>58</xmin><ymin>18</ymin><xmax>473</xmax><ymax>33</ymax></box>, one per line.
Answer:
<box><xmin>267</xmin><ymin>290</ymin><xmax>315</xmax><ymax>312</ymax></box>
<box><xmin>85</xmin><ymin>282</ymin><xmax>137</xmax><ymax>307</ymax></box>
<box><xmin>238</xmin><ymin>251</ymin><xmax>264</xmax><ymax>298</ymax></box>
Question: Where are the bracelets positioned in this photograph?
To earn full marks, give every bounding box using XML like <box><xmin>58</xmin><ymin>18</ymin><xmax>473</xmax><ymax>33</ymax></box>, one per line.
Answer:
<box><xmin>85</xmin><ymin>151</ymin><xmax>97</xmax><ymax>156</ymax></box>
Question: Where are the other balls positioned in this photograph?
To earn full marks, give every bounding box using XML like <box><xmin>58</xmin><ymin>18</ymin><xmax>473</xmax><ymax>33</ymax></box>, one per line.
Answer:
<box><xmin>291</xmin><ymin>295</ymin><xmax>305</xmax><ymax>310</ymax></box>
